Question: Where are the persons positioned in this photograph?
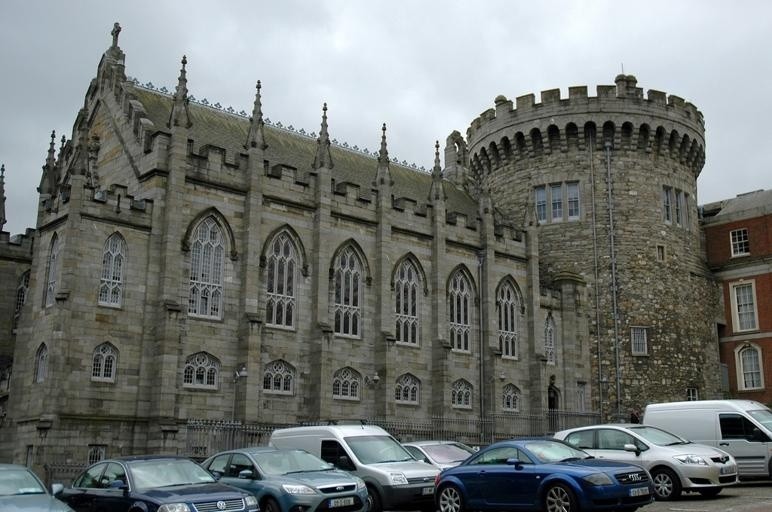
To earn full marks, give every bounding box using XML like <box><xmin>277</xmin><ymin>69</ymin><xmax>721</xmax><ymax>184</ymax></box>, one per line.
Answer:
<box><xmin>630</xmin><ymin>407</ymin><xmax>641</xmax><ymax>424</ymax></box>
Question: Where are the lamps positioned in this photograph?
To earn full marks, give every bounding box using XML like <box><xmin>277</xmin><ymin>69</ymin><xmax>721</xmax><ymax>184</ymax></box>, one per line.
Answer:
<box><xmin>490</xmin><ymin>371</ymin><xmax>506</xmax><ymax>384</ymax></box>
<box><xmin>366</xmin><ymin>370</ymin><xmax>380</xmax><ymax>385</ymax></box>
<box><xmin>233</xmin><ymin>362</ymin><xmax>248</xmax><ymax>384</ymax></box>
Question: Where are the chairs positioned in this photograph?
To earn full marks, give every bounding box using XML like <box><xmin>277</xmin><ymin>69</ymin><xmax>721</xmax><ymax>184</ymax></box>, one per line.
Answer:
<box><xmin>584</xmin><ymin>434</ymin><xmax>633</xmax><ymax>451</ymax></box>
<box><xmin>225</xmin><ymin>455</ymin><xmax>288</xmax><ymax>480</ymax></box>
